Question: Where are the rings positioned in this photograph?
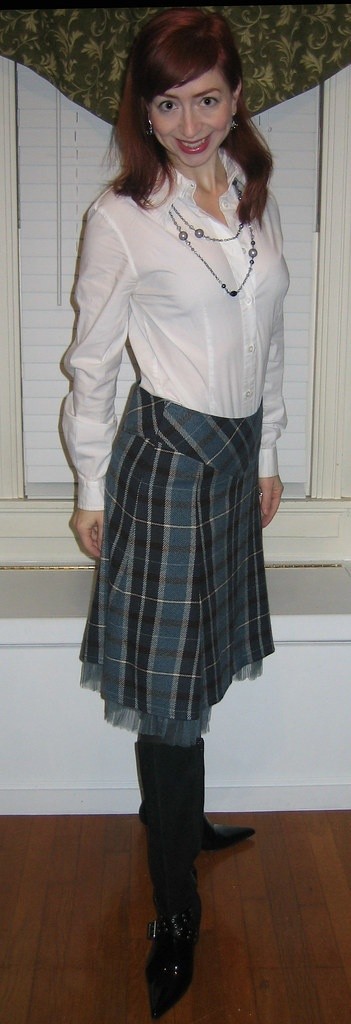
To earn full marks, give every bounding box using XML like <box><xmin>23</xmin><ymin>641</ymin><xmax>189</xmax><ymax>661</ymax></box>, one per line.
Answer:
<box><xmin>259</xmin><ymin>492</ymin><xmax>262</xmax><ymax>496</ymax></box>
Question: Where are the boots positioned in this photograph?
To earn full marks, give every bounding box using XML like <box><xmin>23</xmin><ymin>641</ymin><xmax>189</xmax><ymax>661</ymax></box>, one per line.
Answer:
<box><xmin>192</xmin><ymin>737</ymin><xmax>255</xmax><ymax>853</ymax></box>
<box><xmin>134</xmin><ymin>741</ymin><xmax>201</xmax><ymax>1019</ymax></box>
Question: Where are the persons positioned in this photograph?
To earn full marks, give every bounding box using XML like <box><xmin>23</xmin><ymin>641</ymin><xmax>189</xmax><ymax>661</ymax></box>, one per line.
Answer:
<box><xmin>61</xmin><ymin>4</ymin><xmax>290</xmax><ymax>1006</ymax></box>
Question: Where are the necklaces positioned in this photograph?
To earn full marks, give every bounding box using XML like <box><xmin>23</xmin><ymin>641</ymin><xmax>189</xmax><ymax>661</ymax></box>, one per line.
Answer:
<box><xmin>169</xmin><ymin>181</ymin><xmax>258</xmax><ymax>297</ymax></box>
